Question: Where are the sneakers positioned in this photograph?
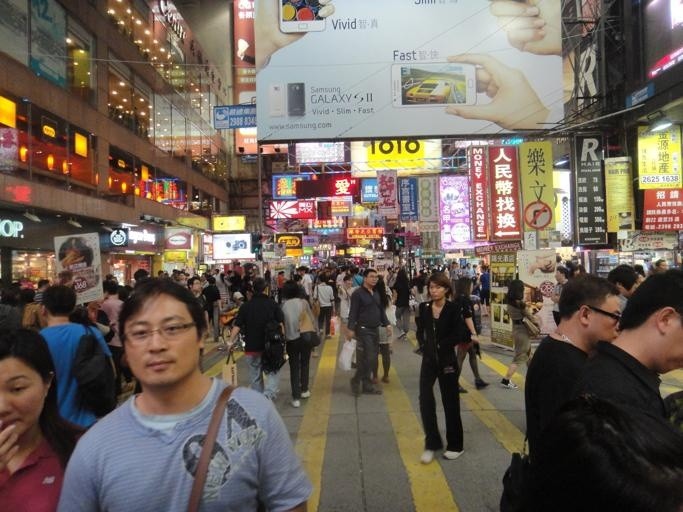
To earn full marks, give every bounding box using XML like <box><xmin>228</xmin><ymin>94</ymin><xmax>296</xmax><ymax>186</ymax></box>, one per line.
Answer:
<box><xmin>420</xmin><ymin>449</ymin><xmax>436</xmax><ymax>464</ymax></box>
<box><xmin>291</xmin><ymin>399</ymin><xmax>301</xmax><ymax>408</ymax></box>
<box><xmin>300</xmin><ymin>390</ymin><xmax>311</xmax><ymax>398</ymax></box>
<box><xmin>499</xmin><ymin>377</ymin><xmax>519</xmax><ymax>390</ymax></box>
<box><xmin>443</xmin><ymin>448</ymin><xmax>465</xmax><ymax>460</ymax></box>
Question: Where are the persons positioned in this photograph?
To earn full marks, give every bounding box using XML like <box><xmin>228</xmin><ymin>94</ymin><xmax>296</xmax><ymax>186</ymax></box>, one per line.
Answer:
<box><xmin>414</xmin><ymin>271</ymin><xmax>464</xmax><ymax>464</ymax></box>
<box><xmin>442</xmin><ymin>52</ymin><xmax>565</xmax><ymax>130</ymax></box>
<box><xmin>1</xmin><ymin>262</ymin><xmax>312</xmax><ymax>512</ymax></box>
<box><xmin>489</xmin><ymin>0</ymin><xmax>562</xmax><ymax>56</ymax></box>
<box><xmin>227</xmin><ymin>261</ymin><xmax>495</xmax><ymax>398</ymax></box>
<box><xmin>501</xmin><ymin>259</ymin><xmax>683</xmax><ymax>512</ymax></box>
<box><xmin>253</xmin><ymin>0</ymin><xmax>335</xmax><ymax>75</ymax></box>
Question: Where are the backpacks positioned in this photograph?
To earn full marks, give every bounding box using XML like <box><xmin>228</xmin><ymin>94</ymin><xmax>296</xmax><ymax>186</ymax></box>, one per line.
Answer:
<box><xmin>261</xmin><ymin>319</ymin><xmax>287</xmax><ymax>375</ymax></box>
<box><xmin>71</xmin><ymin>332</ymin><xmax>117</xmax><ymax>419</ymax></box>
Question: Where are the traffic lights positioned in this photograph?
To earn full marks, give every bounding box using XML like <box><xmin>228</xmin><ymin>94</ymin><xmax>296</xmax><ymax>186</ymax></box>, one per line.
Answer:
<box><xmin>394</xmin><ymin>236</ymin><xmax>405</xmax><ymax>247</ymax></box>
<box><xmin>251</xmin><ymin>231</ymin><xmax>262</xmax><ymax>254</ymax></box>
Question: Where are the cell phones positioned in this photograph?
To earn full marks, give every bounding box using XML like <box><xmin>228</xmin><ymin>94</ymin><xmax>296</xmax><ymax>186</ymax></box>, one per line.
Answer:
<box><xmin>287</xmin><ymin>83</ymin><xmax>306</xmax><ymax>116</ymax></box>
<box><xmin>392</xmin><ymin>61</ymin><xmax>477</xmax><ymax>108</ymax></box>
<box><xmin>269</xmin><ymin>84</ymin><xmax>285</xmax><ymax>119</ymax></box>
<box><xmin>279</xmin><ymin>1</ymin><xmax>327</xmax><ymax>33</ymax></box>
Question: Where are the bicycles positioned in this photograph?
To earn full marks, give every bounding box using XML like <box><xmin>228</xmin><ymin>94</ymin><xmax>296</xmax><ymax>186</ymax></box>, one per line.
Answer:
<box><xmin>219</xmin><ymin>313</ymin><xmax>241</xmax><ymax>348</ymax></box>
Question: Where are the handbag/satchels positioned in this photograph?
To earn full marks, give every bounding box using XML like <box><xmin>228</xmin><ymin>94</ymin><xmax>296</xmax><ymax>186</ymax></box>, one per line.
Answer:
<box><xmin>522</xmin><ymin>316</ymin><xmax>541</xmax><ymax>337</ymax></box>
<box><xmin>497</xmin><ymin>451</ymin><xmax>541</xmax><ymax>511</ymax></box>
<box><xmin>222</xmin><ymin>350</ymin><xmax>238</xmax><ymax>387</ymax></box>
<box><xmin>298</xmin><ymin>307</ymin><xmax>320</xmax><ymax>351</ymax></box>
<box><xmin>338</xmin><ymin>339</ymin><xmax>357</xmax><ymax>374</ymax></box>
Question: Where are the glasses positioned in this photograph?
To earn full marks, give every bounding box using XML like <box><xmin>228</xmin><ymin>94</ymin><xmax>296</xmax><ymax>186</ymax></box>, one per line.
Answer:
<box><xmin>123</xmin><ymin>321</ymin><xmax>199</xmax><ymax>344</ymax></box>
<box><xmin>587</xmin><ymin>304</ymin><xmax>621</xmax><ymax>323</ymax></box>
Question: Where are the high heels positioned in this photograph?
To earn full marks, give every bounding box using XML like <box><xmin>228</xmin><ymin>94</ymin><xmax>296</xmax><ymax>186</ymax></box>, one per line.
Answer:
<box><xmin>475</xmin><ymin>379</ymin><xmax>490</xmax><ymax>390</ymax></box>
